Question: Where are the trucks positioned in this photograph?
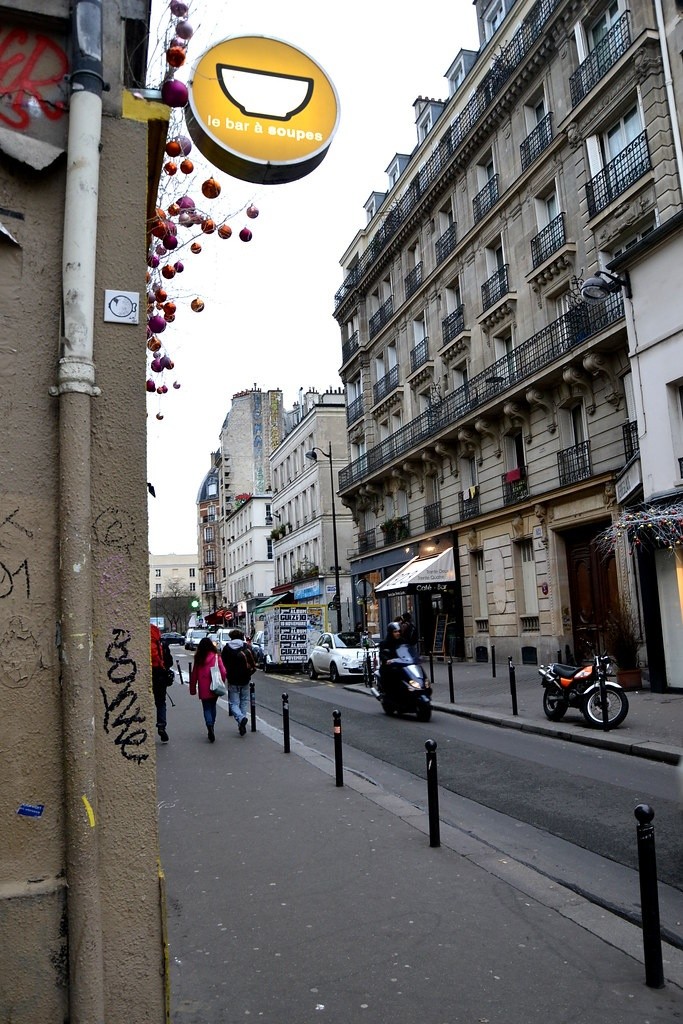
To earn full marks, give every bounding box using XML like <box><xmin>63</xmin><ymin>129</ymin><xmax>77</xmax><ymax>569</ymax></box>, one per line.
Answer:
<box><xmin>249</xmin><ymin>603</ymin><xmax>329</xmax><ymax>675</ymax></box>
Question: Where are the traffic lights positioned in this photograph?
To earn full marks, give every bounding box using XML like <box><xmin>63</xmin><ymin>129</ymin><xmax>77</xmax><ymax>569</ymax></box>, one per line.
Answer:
<box><xmin>191</xmin><ymin>600</ymin><xmax>199</xmax><ymax>609</ymax></box>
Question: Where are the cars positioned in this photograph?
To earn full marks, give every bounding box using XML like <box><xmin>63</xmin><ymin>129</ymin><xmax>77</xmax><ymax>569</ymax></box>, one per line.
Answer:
<box><xmin>308</xmin><ymin>631</ymin><xmax>380</xmax><ymax>683</ymax></box>
<box><xmin>182</xmin><ymin>628</ymin><xmax>237</xmax><ymax>652</ymax></box>
<box><xmin>160</xmin><ymin>632</ymin><xmax>185</xmax><ymax>646</ymax></box>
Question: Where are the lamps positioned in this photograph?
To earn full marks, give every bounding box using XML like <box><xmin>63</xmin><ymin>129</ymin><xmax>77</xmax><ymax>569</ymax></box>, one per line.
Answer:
<box><xmin>305</xmin><ymin>446</ymin><xmax>330</xmax><ymax>465</ymax></box>
<box><xmin>580</xmin><ymin>270</ymin><xmax>632</xmax><ymax>307</ymax></box>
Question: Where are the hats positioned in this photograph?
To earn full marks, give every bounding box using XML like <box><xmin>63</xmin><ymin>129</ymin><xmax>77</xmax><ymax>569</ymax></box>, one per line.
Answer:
<box><xmin>401</xmin><ymin>612</ymin><xmax>411</xmax><ymax>619</ymax></box>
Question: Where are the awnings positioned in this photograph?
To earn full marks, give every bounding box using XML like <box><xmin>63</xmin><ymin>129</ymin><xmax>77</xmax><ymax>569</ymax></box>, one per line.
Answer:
<box><xmin>255</xmin><ymin>592</ymin><xmax>288</xmax><ymax>614</ymax></box>
<box><xmin>205</xmin><ymin>613</ymin><xmax>224</xmax><ymax>625</ymax></box>
<box><xmin>374</xmin><ymin>546</ymin><xmax>457</xmax><ymax>598</ymax></box>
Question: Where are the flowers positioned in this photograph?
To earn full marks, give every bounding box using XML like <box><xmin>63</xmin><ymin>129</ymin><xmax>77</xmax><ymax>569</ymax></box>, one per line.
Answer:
<box><xmin>234</xmin><ymin>492</ymin><xmax>253</xmax><ymax>509</ymax></box>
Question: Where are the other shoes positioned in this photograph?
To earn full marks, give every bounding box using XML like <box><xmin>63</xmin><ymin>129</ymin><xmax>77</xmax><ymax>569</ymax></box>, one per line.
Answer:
<box><xmin>207</xmin><ymin>726</ymin><xmax>215</xmax><ymax>743</ymax></box>
<box><xmin>239</xmin><ymin>717</ymin><xmax>248</xmax><ymax>736</ymax></box>
<box><xmin>157</xmin><ymin>728</ymin><xmax>170</xmax><ymax>743</ymax></box>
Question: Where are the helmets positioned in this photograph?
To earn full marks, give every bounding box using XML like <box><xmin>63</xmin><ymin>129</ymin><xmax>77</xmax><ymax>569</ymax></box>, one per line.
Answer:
<box><xmin>387</xmin><ymin>622</ymin><xmax>401</xmax><ymax>637</ymax></box>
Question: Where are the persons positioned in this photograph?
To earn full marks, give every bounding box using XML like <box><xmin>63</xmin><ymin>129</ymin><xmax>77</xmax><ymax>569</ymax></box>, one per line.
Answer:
<box><xmin>221</xmin><ymin>631</ymin><xmax>255</xmax><ymax>735</ymax></box>
<box><xmin>380</xmin><ymin>613</ymin><xmax>417</xmax><ymax>678</ymax></box>
<box><xmin>190</xmin><ymin>638</ymin><xmax>227</xmax><ymax>743</ymax></box>
<box><xmin>151</xmin><ymin>624</ymin><xmax>169</xmax><ymax>742</ymax></box>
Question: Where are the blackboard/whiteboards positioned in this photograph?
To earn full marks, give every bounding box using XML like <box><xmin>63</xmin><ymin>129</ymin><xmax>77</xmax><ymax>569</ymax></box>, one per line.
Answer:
<box><xmin>433</xmin><ymin>614</ymin><xmax>447</xmax><ymax>652</ymax></box>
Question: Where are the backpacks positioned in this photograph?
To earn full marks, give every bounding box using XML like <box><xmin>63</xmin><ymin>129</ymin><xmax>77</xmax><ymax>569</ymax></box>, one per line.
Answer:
<box><xmin>238</xmin><ymin>647</ymin><xmax>257</xmax><ymax>678</ymax></box>
<box><xmin>405</xmin><ymin>622</ymin><xmax>418</xmax><ymax>646</ymax></box>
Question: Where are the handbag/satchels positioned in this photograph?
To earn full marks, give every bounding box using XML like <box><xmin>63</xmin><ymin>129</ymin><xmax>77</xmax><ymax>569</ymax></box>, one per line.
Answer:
<box><xmin>210</xmin><ymin>653</ymin><xmax>226</xmax><ymax>696</ymax></box>
<box><xmin>164</xmin><ymin>669</ymin><xmax>175</xmax><ymax>686</ymax></box>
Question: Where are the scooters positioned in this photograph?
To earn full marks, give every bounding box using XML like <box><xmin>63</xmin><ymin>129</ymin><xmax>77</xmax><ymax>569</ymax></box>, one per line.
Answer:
<box><xmin>373</xmin><ymin>637</ymin><xmax>434</xmax><ymax>724</ymax></box>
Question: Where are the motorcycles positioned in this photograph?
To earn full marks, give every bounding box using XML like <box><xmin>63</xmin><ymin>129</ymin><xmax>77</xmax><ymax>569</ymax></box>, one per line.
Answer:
<box><xmin>538</xmin><ymin>640</ymin><xmax>630</xmax><ymax>732</ymax></box>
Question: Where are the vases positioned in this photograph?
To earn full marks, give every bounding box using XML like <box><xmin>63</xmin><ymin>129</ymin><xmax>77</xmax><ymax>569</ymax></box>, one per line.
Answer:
<box><xmin>616</xmin><ymin>669</ymin><xmax>642</xmax><ymax>690</ymax></box>
